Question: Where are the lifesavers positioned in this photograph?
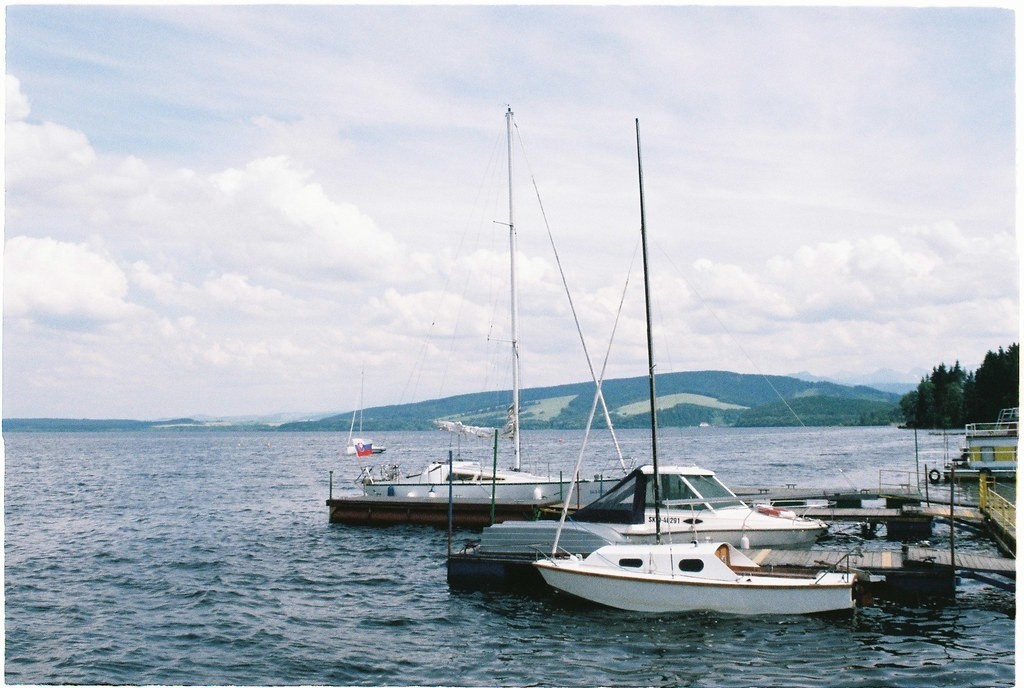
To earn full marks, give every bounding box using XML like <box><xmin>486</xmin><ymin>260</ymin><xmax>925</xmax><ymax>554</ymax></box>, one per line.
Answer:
<box><xmin>756</xmin><ymin>504</ymin><xmax>797</xmax><ymax>520</ymax></box>
<box><xmin>929</xmin><ymin>469</ymin><xmax>941</xmax><ymax>482</ymax></box>
<box><xmin>854</xmin><ymin>581</ymin><xmax>868</xmax><ymax>596</ymax></box>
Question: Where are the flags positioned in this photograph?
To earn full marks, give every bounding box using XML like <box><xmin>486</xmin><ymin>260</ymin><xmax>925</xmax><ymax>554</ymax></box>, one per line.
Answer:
<box><xmin>354</xmin><ymin>439</ymin><xmax>373</xmax><ymax>457</ymax></box>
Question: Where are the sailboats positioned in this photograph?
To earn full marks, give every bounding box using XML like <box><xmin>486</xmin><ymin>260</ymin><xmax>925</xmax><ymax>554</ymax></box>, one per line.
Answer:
<box><xmin>443</xmin><ymin>119</ymin><xmax>827</xmax><ymax>591</ymax></box>
<box><xmin>325</xmin><ymin>105</ymin><xmax>632</xmax><ymax>530</ymax></box>
<box><xmin>345</xmin><ymin>368</ymin><xmax>387</xmax><ymax>454</ymax></box>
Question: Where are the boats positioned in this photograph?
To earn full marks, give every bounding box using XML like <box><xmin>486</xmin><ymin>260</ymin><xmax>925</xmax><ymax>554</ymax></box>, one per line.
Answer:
<box><xmin>944</xmin><ymin>405</ymin><xmax>1019</xmax><ymax>480</ymax></box>
<box><xmin>530</xmin><ymin>538</ymin><xmax>862</xmax><ymax>615</ymax></box>
